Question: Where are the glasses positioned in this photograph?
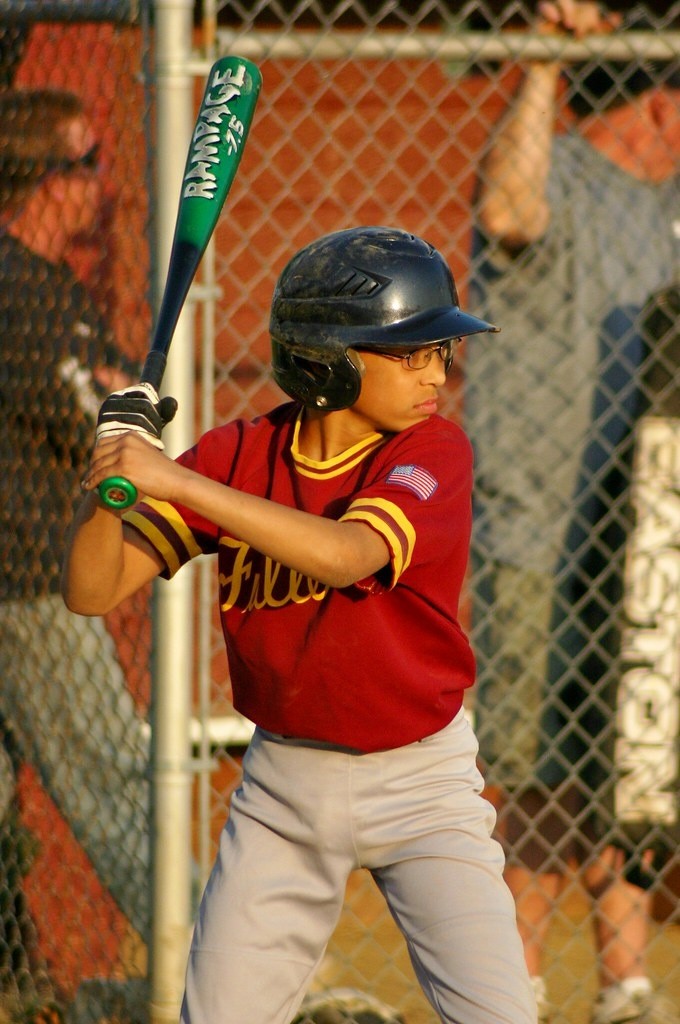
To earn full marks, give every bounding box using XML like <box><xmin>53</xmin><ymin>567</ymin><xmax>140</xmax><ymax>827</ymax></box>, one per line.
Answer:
<box><xmin>47</xmin><ymin>144</ymin><xmax>100</xmax><ymax>171</ymax></box>
<box><xmin>349</xmin><ymin>338</ymin><xmax>463</xmax><ymax>369</ymax></box>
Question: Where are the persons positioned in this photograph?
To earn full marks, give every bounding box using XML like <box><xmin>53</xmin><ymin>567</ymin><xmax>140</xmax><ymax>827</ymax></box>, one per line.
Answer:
<box><xmin>0</xmin><ymin>88</ymin><xmax>207</xmax><ymax>1024</ymax></box>
<box><xmin>462</xmin><ymin>1</ymin><xmax>680</xmax><ymax>1024</ymax></box>
<box><xmin>62</xmin><ymin>224</ymin><xmax>543</xmax><ymax>1024</ymax></box>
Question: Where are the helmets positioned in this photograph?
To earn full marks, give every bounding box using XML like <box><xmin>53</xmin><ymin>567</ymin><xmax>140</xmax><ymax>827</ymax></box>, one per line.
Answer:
<box><xmin>268</xmin><ymin>225</ymin><xmax>502</xmax><ymax>411</ymax></box>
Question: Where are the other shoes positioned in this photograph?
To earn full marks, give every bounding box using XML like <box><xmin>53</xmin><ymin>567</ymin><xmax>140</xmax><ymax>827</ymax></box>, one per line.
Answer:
<box><xmin>586</xmin><ymin>976</ymin><xmax>656</xmax><ymax>1024</ymax></box>
<box><xmin>530</xmin><ymin>976</ymin><xmax>550</xmax><ymax>1019</ymax></box>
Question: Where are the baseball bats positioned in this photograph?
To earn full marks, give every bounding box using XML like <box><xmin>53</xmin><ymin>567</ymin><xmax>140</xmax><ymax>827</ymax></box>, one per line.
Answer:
<box><xmin>101</xmin><ymin>54</ymin><xmax>265</xmax><ymax>509</ymax></box>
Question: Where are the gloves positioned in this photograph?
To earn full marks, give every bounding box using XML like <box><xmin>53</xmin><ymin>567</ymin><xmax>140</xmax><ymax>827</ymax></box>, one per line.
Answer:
<box><xmin>95</xmin><ymin>382</ymin><xmax>177</xmax><ymax>450</ymax></box>
